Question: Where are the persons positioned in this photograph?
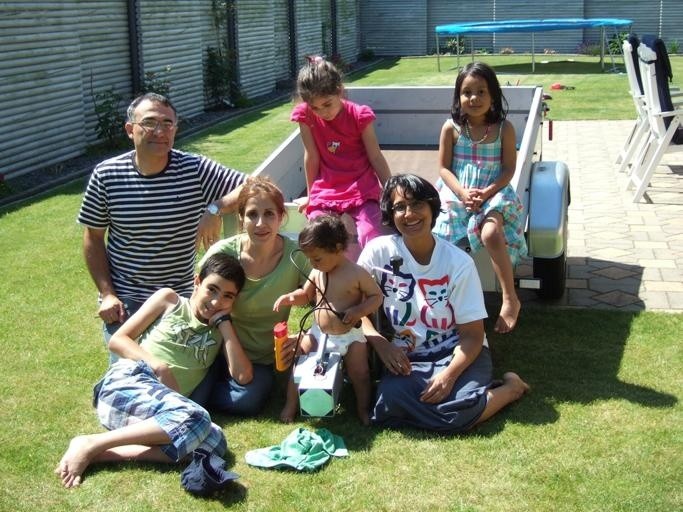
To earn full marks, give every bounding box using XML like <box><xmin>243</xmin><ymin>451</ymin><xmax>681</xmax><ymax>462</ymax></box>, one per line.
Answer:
<box><xmin>76</xmin><ymin>92</ymin><xmax>251</xmax><ymax>366</ymax></box>
<box><xmin>356</xmin><ymin>173</ymin><xmax>531</xmax><ymax>434</ymax></box>
<box><xmin>55</xmin><ymin>252</ymin><xmax>254</xmax><ymax>488</ymax></box>
<box><xmin>272</xmin><ymin>215</ymin><xmax>384</xmax><ymax>426</ymax></box>
<box><xmin>193</xmin><ymin>180</ymin><xmax>314</xmax><ymax>416</ymax></box>
<box><xmin>431</xmin><ymin>61</ymin><xmax>528</xmax><ymax>335</ymax></box>
<box><xmin>290</xmin><ymin>56</ymin><xmax>392</xmax><ymax>251</ymax></box>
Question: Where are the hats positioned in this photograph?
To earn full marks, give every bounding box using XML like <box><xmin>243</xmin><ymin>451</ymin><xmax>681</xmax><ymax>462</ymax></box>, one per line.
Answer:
<box><xmin>181</xmin><ymin>448</ymin><xmax>240</xmax><ymax>495</ymax></box>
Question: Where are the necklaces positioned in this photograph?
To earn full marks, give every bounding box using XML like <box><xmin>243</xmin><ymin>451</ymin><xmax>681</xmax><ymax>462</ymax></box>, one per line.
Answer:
<box><xmin>465</xmin><ymin>121</ymin><xmax>490</xmax><ymax>145</ymax></box>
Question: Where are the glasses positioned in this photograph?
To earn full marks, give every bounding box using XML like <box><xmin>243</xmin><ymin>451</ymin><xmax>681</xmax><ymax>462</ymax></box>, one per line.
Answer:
<box><xmin>131</xmin><ymin>120</ymin><xmax>176</xmax><ymax>130</ymax></box>
<box><xmin>391</xmin><ymin>198</ymin><xmax>424</xmax><ymax>212</ymax></box>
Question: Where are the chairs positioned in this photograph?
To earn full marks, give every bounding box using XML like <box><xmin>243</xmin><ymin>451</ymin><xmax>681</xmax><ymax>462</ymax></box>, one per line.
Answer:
<box><xmin>625</xmin><ymin>34</ymin><xmax>683</xmax><ymax>202</ymax></box>
<box><xmin>615</xmin><ymin>34</ymin><xmax>683</xmax><ymax>172</ymax></box>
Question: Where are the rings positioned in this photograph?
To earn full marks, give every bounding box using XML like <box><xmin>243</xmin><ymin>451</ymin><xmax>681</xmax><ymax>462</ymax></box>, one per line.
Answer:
<box><xmin>292</xmin><ymin>347</ymin><xmax>296</xmax><ymax>352</ymax></box>
<box><xmin>294</xmin><ymin>354</ymin><xmax>298</xmax><ymax>359</ymax></box>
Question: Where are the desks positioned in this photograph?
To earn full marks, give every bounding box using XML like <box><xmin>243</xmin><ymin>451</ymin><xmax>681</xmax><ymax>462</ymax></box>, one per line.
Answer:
<box><xmin>434</xmin><ymin>16</ymin><xmax>635</xmax><ymax>74</ymax></box>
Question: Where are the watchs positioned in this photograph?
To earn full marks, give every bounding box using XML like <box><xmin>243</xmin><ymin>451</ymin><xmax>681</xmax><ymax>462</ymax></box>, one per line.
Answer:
<box><xmin>204</xmin><ymin>202</ymin><xmax>223</xmax><ymax>218</ymax></box>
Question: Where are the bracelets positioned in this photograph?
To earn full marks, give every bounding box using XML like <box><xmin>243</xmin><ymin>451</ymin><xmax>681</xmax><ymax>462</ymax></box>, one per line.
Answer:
<box><xmin>213</xmin><ymin>314</ymin><xmax>233</xmax><ymax>329</ymax></box>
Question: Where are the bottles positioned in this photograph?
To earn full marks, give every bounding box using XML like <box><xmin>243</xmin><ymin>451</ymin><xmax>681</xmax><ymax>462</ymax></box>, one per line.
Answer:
<box><xmin>273</xmin><ymin>322</ymin><xmax>292</xmax><ymax>372</ymax></box>
